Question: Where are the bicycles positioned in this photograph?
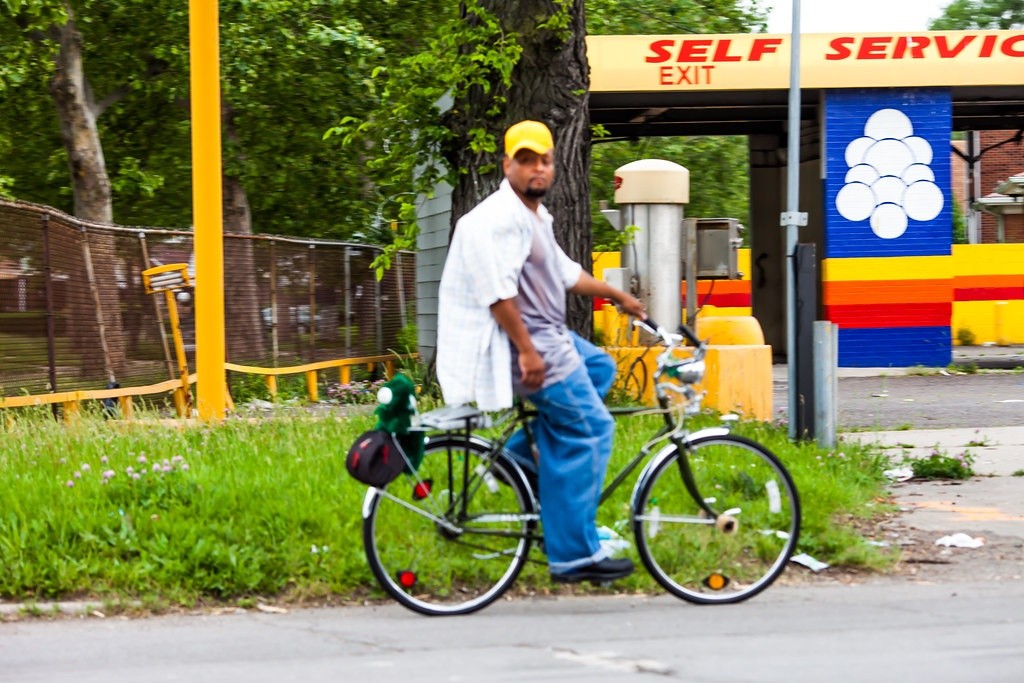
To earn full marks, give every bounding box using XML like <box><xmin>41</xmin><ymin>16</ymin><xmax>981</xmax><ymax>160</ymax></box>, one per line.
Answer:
<box><xmin>360</xmin><ymin>315</ymin><xmax>802</xmax><ymax>617</ymax></box>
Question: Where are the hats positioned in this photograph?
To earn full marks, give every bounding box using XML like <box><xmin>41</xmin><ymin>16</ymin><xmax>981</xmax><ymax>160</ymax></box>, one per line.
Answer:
<box><xmin>505</xmin><ymin>119</ymin><xmax>554</xmax><ymax>159</ymax></box>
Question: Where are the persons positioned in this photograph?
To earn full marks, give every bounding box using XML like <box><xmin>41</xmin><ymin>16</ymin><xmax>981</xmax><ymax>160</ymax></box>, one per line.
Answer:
<box><xmin>436</xmin><ymin>119</ymin><xmax>648</xmax><ymax>583</ymax></box>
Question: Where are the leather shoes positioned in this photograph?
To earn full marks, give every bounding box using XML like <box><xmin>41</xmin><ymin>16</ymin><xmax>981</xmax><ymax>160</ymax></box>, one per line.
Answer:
<box><xmin>551</xmin><ymin>556</ymin><xmax>634</xmax><ymax>582</ymax></box>
<box><xmin>488</xmin><ymin>450</ymin><xmax>541</xmax><ymax>501</ymax></box>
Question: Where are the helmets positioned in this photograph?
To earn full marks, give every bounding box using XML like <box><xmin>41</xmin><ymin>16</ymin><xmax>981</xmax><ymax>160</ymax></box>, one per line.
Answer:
<box><xmin>346</xmin><ymin>430</ymin><xmax>403</xmax><ymax>488</ymax></box>
